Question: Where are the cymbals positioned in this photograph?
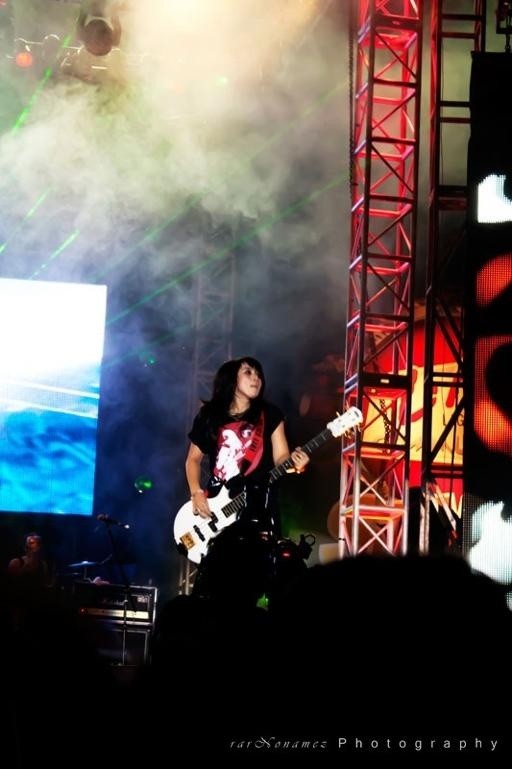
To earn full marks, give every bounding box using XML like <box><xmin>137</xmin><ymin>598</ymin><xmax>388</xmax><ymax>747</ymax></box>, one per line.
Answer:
<box><xmin>69</xmin><ymin>561</ymin><xmax>96</xmax><ymax>568</ymax></box>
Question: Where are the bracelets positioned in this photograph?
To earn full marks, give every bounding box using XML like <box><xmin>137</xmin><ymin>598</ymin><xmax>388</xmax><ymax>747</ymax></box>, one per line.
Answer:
<box><xmin>190</xmin><ymin>489</ymin><xmax>205</xmax><ymax>499</ymax></box>
<box><xmin>294</xmin><ymin>467</ymin><xmax>306</xmax><ymax>475</ymax></box>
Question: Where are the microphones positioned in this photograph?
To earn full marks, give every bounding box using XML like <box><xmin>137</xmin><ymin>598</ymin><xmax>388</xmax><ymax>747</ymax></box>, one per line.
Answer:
<box><xmin>96</xmin><ymin>512</ymin><xmax>132</xmax><ymax>530</ymax></box>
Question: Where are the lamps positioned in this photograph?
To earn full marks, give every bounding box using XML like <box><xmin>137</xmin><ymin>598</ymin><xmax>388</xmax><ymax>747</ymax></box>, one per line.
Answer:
<box><xmin>73</xmin><ymin>0</ymin><xmax>122</xmax><ymax>57</ymax></box>
<box><xmin>14</xmin><ymin>40</ymin><xmax>34</xmax><ymax>69</ymax></box>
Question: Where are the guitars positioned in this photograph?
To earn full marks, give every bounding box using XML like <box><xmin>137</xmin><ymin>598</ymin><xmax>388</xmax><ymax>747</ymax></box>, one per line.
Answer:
<box><xmin>173</xmin><ymin>405</ymin><xmax>364</xmax><ymax>564</ymax></box>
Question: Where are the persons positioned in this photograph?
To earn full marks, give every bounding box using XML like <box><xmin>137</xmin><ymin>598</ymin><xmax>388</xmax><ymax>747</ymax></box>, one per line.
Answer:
<box><xmin>2</xmin><ymin>529</ymin><xmax>512</xmax><ymax>768</ymax></box>
<box><xmin>184</xmin><ymin>355</ymin><xmax>314</xmax><ymax>571</ymax></box>
